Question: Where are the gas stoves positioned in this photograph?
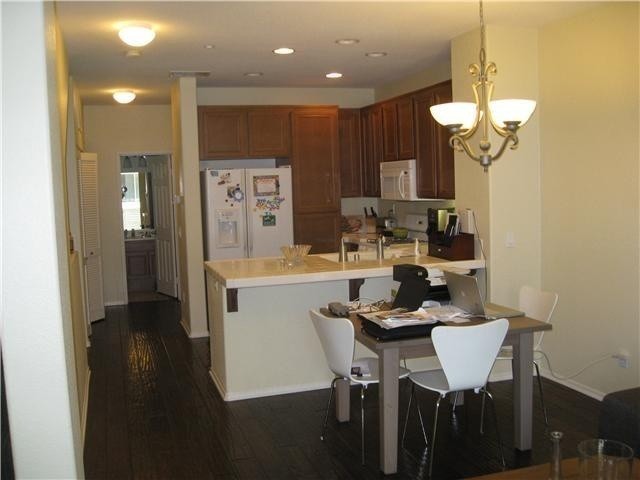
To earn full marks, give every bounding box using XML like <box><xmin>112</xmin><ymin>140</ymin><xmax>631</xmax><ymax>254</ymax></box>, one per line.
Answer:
<box><xmin>361</xmin><ymin>229</ymin><xmax>429</xmax><ymax>251</ymax></box>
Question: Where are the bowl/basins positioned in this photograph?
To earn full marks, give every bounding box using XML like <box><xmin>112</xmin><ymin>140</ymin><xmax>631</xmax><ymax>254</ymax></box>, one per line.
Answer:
<box><xmin>280</xmin><ymin>244</ymin><xmax>312</xmax><ymax>260</ymax></box>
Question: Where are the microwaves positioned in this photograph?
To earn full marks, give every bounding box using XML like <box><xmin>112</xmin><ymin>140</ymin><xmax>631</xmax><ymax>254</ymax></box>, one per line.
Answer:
<box><xmin>380</xmin><ymin>160</ymin><xmax>416</xmax><ymax>201</ymax></box>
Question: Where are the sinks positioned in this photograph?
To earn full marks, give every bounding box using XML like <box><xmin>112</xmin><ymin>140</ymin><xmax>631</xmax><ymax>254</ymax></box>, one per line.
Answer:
<box><xmin>319</xmin><ymin>249</ymin><xmax>415</xmax><ymax>263</ymax></box>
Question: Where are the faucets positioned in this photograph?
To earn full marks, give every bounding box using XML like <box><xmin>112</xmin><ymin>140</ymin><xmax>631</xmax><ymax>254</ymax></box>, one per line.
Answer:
<box><xmin>367</xmin><ymin>236</ymin><xmax>384</xmax><ymax>259</ymax></box>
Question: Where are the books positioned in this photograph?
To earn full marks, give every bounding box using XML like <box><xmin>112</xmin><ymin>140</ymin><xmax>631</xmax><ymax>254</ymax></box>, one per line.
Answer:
<box><xmin>424</xmin><ymin>305</ymin><xmax>467</xmax><ymax>324</ymax></box>
<box><xmin>380</xmin><ymin>307</ymin><xmax>436</xmax><ymax>330</ymax></box>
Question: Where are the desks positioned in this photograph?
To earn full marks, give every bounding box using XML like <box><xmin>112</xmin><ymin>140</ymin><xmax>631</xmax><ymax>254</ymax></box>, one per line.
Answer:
<box><xmin>319</xmin><ymin>301</ymin><xmax>553</xmax><ymax>475</ymax></box>
<box><xmin>465</xmin><ymin>455</ymin><xmax>640</xmax><ymax>479</ymax></box>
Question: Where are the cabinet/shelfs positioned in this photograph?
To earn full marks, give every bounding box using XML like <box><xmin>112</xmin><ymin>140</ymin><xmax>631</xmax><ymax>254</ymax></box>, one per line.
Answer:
<box><xmin>198</xmin><ymin>106</ymin><xmax>290</xmax><ymax>162</ymax></box>
<box><xmin>339</xmin><ymin>108</ymin><xmax>361</xmax><ymax>197</ymax></box>
<box><xmin>291</xmin><ymin>104</ymin><xmax>341</xmax><ymax>214</ymax></box>
<box><xmin>124</xmin><ymin>240</ymin><xmax>157</xmax><ymax>292</ymax></box>
<box><xmin>361</xmin><ymin>79</ymin><xmax>456</xmax><ymax>199</ymax></box>
<box><xmin>293</xmin><ymin>212</ymin><xmax>342</xmax><ymax>255</ymax></box>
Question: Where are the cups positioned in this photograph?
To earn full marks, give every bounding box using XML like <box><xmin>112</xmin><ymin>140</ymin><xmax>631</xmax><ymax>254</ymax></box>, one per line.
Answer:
<box><xmin>578</xmin><ymin>438</ymin><xmax>633</xmax><ymax>480</ymax></box>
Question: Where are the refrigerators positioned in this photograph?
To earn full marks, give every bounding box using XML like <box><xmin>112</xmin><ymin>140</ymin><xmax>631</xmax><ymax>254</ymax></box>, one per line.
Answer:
<box><xmin>205</xmin><ymin>166</ymin><xmax>292</xmax><ymax>259</ymax></box>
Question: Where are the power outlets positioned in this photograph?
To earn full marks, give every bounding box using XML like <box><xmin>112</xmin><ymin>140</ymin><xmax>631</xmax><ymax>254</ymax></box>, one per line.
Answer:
<box><xmin>616</xmin><ymin>353</ymin><xmax>630</xmax><ymax>368</ymax></box>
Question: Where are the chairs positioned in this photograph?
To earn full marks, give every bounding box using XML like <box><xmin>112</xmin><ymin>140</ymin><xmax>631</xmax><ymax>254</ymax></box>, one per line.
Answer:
<box><xmin>309</xmin><ymin>308</ymin><xmax>428</xmax><ymax>466</ymax></box>
<box><xmin>480</xmin><ymin>286</ymin><xmax>559</xmax><ymax>433</ymax></box>
<box><xmin>402</xmin><ymin>318</ymin><xmax>509</xmax><ymax>480</ymax></box>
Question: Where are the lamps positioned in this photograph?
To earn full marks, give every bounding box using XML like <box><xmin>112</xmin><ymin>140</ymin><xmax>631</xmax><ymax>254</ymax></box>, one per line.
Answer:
<box><xmin>428</xmin><ymin>2</ymin><xmax>537</xmax><ymax>175</ymax></box>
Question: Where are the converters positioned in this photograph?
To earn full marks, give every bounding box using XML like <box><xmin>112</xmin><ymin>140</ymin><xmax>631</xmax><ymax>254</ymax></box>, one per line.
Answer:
<box><xmin>329</xmin><ymin>302</ymin><xmax>348</xmax><ymax>317</ymax></box>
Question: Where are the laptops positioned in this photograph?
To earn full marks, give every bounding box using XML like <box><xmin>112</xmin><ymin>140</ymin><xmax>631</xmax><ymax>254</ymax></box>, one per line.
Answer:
<box><xmin>357</xmin><ymin>274</ymin><xmax>431</xmax><ymax>322</ymax></box>
<box><xmin>444</xmin><ymin>269</ymin><xmax>526</xmax><ymax>319</ymax></box>
